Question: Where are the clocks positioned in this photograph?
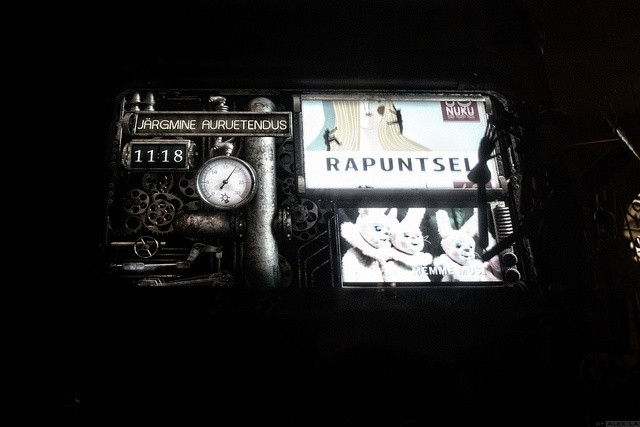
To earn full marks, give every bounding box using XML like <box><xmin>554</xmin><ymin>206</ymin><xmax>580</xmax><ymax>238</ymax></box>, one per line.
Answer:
<box><xmin>196</xmin><ymin>156</ymin><xmax>256</xmax><ymax>209</ymax></box>
